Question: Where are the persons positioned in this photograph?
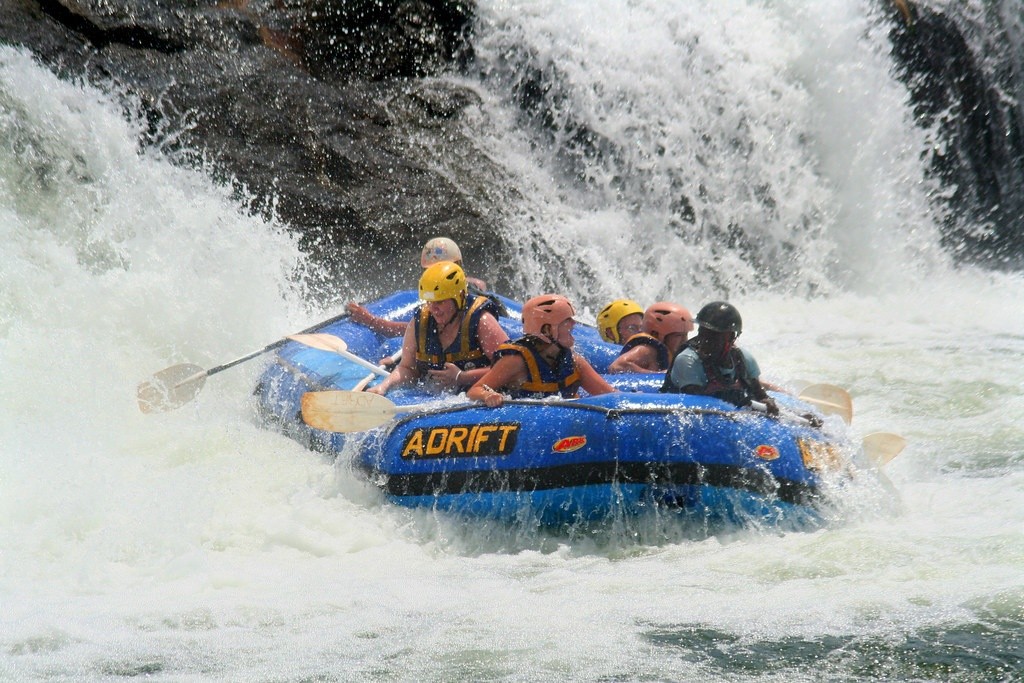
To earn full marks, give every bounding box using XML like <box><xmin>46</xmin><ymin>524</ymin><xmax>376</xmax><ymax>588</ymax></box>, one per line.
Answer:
<box><xmin>366</xmin><ymin>262</ymin><xmax>514</xmax><ymax>395</ymax></box>
<box><xmin>596</xmin><ymin>300</ymin><xmax>645</xmax><ymax>345</ymax></box>
<box><xmin>465</xmin><ymin>294</ymin><xmax>622</xmax><ymax>408</ymax></box>
<box><xmin>345</xmin><ymin>237</ymin><xmax>496</xmax><ymax>368</ymax></box>
<box><xmin>665</xmin><ymin>302</ymin><xmax>772</xmax><ymax>404</ymax></box>
<box><xmin>607</xmin><ymin>302</ymin><xmax>694</xmax><ymax>375</ymax></box>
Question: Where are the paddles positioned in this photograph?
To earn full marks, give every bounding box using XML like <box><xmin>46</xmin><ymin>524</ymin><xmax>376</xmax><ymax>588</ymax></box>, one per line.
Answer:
<box><xmin>286</xmin><ymin>332</ymin><xmax>391</xmax><ymax>378</ymax></box>
<box><xmin>351</xmin><ymin>350</ymin><xmax>402</xmax><ymax>392</ymax></box>
<box><xmin>293</xmin><ymin>389</ymin><xmax>469</xmax><ymax>434</ymax></box>
<box><xmin>750</xmin><ymin>379</ymin><xmax>909</xmax><ymax>466</ymax></box>
<box><xmin>135</xmin><ymin>313</ymin><xmax>357</xmax><ymax>415</ymax></box>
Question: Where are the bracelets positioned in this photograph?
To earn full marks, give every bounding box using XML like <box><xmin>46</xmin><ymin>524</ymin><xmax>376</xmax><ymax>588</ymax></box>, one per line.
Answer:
<box><xmin>368</xmin><ymin>316</ymin><xmax>376</xmax><ymax>329</ymax></box>
<box><xmin>454</xmin><ymin>370</ymin><xmax>463</xmax><ymax>385</ymax></box>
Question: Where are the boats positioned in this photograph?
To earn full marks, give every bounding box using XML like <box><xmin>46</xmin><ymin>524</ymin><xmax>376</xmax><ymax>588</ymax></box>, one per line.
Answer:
<box><xmin>249</xmin><ymin>291</ymin><xmax>869</xmax><ymax>533</ymax></box>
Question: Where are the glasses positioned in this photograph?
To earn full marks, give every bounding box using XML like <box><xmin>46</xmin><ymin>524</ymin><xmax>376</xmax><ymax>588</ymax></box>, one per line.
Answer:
<box><xmin>623</xmin><ymin>324</ymin><xmax>643</xmax><ymax>334</ymax></box>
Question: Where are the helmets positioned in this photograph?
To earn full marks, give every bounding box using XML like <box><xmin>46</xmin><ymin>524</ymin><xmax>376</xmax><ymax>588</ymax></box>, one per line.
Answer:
<box><xmin>695</xmin><ymin>301</ymin><xmax>742</xmax><ymax>337</ymax></box>
<box><xmin>418</xmin><ymin>262</ymin><xmax>467</xmax><ymax>309</ymax></box>
<box><xmin>421</xmin><ymin>237</ymin><xmax>462</xmax><ymax>271</ymax></box>
<box><xmin>522</xmin><ymin>294</ymin><xmax>574</xmax><ymax>343</ymax></box>
<box><xmin>597</xmin><ymin>300</ymin><xmax>645</xmax><ymax>343</ymax></box>
<box><xmin>643</xmin><ymin>301</ymin><xmax>695</xmax><ymax>342</ymax></box>
